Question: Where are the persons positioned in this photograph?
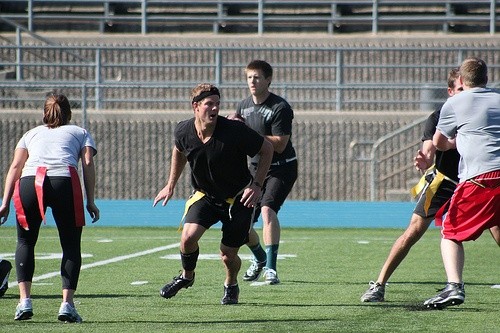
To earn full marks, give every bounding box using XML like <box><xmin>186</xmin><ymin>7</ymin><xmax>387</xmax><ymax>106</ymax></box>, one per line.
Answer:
<box><xmin>0</xmin><ymin>95</ymin><xmax>100</xmax><ymax>322</ymax></box>
<box><xmin>152</xmin><ymin>83</ymin><xmax>275</xmax><ymax>306</ymax></box>
<box><xmin>227</xmin><ymin>60</ymin><xmax>298</xmax><ymax>283</ymax></box>
<box><xmin>423</xmin><ymin>56</ymin><xmax>500</xmax><ymax>309</ymax></box>
<box><xmin>361</xmin><ymin>65</ymin><xmax>500</xmax><ymax>304</ymax></box>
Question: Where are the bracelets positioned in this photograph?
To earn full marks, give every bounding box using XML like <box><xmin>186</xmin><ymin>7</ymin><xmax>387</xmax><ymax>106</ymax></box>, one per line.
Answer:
<box><xmin>254</xmin><ymin>181</ymin><xmax>262</xmax><ymax>187</ymax></box>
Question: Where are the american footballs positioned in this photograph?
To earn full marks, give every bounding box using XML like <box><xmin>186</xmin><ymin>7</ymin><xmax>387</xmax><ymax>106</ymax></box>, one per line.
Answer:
<box><xmin>224</xmin><ymin>113</ymin><xmax>245</xmax><ymax>124</ymax></box>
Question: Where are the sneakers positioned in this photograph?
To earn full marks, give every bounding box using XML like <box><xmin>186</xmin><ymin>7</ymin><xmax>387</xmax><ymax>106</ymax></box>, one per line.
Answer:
<box><xmin>262</xmin><ymin>267</ymin><xmax>279</xmax><ymax>285</ymax></box>
<box><xmin>360</xmin><ymin>281</ymin><xmax>384</xmax><ymax>301</ymax></box>
<box><xmin>243</xmin><ymin>259</ymin><xmax>267</xmax><ymax>281</ymax></box>
<box><xmin>222</xmin><ymin>283</ymin><xmax>240</xmax><ymax>305</ymax></box>
<box><xmin>160</xmin><ymin>270</ymin><xmax>195</xmax><ymax>299</ymax></box>
<box><xmin>57</xmin><ymin>301</ymin><xmax>82</xmax><ymax>322</ymax></box>
<box><xmin>14</xmin><ymin>298</ymin><xmax>34</xmax><ymax>321</ymax></box>
<box><xmin>423</xmin><ymin>282</ymin><xmax>464</xmax><ymax>308</ymax></box>
